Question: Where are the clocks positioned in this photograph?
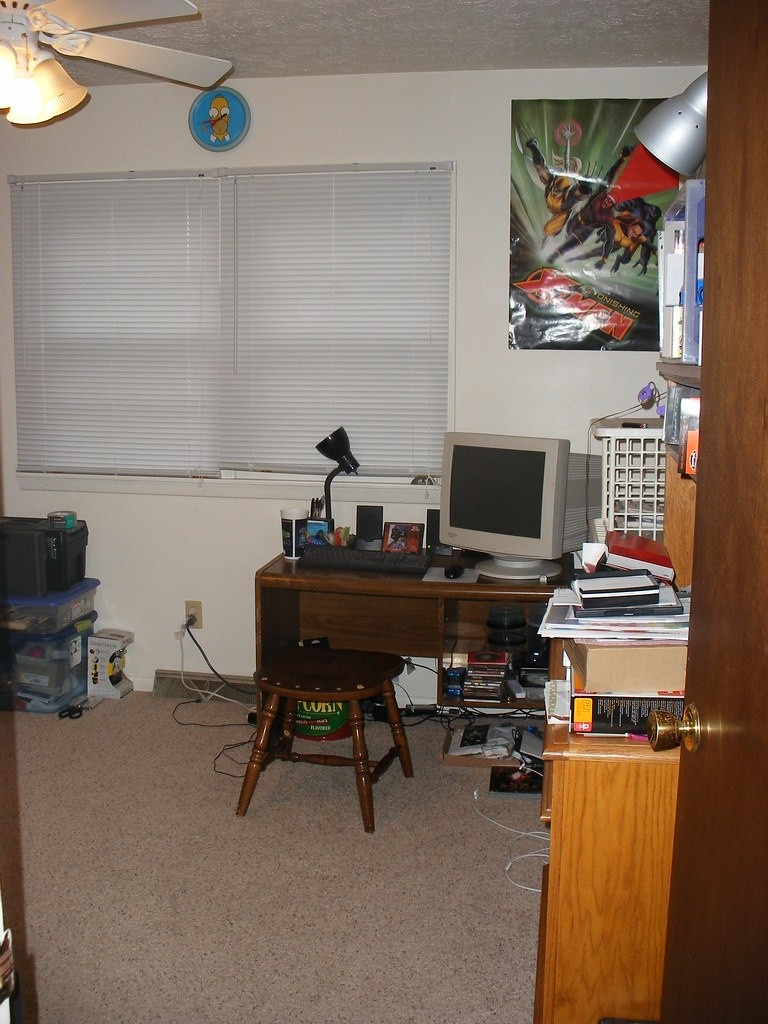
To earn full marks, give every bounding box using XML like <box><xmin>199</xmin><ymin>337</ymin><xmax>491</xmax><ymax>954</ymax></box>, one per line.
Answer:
<box><xmin>187</xmin><ymin>86</ymin><xmax>251</xmax><ymax>152</ymax></box>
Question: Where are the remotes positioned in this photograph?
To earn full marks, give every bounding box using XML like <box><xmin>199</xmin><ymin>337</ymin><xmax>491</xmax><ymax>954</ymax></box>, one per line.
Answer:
<box><xmin>506</xmin><ymin>680</ymin><xmax>526</xmax><ymax>698</ymax></box>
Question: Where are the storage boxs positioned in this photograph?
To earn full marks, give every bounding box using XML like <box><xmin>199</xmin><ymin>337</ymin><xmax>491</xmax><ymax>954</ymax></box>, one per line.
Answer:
<box><xmin>440</xmin><ymin>724</ymin><xmax>522</xmax><ymax>768</ymax></box>
<box><xmin>0</xmin><ymin>516</ymin><xmax>101</xmax><ymax>714</ymax></box>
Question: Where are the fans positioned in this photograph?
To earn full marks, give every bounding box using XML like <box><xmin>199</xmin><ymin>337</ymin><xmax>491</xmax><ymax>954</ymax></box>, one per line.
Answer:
<box><xmin>0</xmin><ymin>0</ymin><xmax>233</xmax><ymax>90</ymax></box>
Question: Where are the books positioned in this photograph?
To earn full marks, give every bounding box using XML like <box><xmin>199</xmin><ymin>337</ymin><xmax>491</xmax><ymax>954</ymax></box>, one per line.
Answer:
<box><xmin>537</xmin><ymin>586</ymin><xmax>691</xmax><ymax>641</ymax></box>
<box><xmin>655</xmin><ymin>215</ymin><xmax>706</xmax><ymax>366</ymax></box>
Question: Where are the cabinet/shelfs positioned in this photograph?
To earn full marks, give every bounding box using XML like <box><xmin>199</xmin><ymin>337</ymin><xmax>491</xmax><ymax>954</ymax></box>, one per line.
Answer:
<box><xmin>254</xmin><ymin>552</ymin><xmax>559</xmax><ymax>735</ymax></box>
<box><xmin>656</xmin><ymin>359</ymin><xmax>701</xmax><ymax>594</ymax></box>
<box><xmin>532</xmin><ymin>637</ymin><xmax>681</xmax><ymax>1024</ymax></box>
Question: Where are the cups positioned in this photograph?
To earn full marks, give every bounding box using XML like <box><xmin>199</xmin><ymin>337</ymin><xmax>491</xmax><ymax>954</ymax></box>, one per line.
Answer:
<box><xmin>281</xmin><ymin>508</ymin><xmax>309</xmax><ymax>560</ymax></box>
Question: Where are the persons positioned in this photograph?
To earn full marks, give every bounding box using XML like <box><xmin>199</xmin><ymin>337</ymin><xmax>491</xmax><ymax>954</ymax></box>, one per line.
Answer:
<box><xmin>389</xmin><ymin>525</ymin><xmax>407</xmax><ymax>548</ymax></box>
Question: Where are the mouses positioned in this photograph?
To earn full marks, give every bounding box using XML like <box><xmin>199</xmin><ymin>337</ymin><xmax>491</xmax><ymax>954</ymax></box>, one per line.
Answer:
<box><xmin>444</xmin><ymin>565</ymin><xmax>464</xmax><ymax>579</ymax></box>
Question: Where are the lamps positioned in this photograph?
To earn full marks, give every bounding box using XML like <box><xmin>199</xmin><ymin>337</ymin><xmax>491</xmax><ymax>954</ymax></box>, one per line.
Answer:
<box><xmin>1</xmin><ymin>38</ymin><xmax>87</xmax><ymax>124</ymax></box>
<box><xmin>315</xmin><ymin>427</ymin><xmax>360</xmax><ymax>519</ymax></box>
<box><xmin>633</xmin><ymin>68</ymin><xmax>708</xmax><ymax>178</ymax></box>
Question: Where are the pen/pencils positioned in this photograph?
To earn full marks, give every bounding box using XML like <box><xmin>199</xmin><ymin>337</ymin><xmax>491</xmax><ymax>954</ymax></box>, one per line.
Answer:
<box><xmin>310</xmin><ymin>495</ymin><xmax>324</xmax><ymax>518</ymax></box>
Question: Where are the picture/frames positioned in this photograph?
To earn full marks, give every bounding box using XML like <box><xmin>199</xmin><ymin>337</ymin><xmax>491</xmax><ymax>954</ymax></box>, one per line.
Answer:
<box><xmin>381</xmin><ymin>521</ymin><xmax>424</xmax><ymax>554</ymax></box>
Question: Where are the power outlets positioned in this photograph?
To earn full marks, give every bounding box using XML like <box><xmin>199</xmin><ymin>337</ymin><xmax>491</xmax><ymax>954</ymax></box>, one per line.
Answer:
<box><xmin>185</xmin><ymin>601</ymin><xmax>203</xmax><ymax>629</ymax></box>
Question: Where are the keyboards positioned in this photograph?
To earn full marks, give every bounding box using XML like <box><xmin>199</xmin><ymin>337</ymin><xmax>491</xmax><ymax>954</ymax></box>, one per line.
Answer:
<box><xmin>295</xmin><ymin>546</ymin><xmax>432</xmax><ymax>575</ymax></box>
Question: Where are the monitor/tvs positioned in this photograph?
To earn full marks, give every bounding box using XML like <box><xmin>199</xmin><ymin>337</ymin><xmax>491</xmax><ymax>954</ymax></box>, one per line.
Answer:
<box><xmin>439</xmin><ymin>432</ymin><xmax>601</xmax><ymax>579</ymax></box>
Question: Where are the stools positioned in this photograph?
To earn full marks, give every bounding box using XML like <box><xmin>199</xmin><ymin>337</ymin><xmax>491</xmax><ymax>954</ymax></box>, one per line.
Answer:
<box><xmin>236</xmin><ymin>647</ymin><xmax>414</xmax><ymax>833</ymax></box>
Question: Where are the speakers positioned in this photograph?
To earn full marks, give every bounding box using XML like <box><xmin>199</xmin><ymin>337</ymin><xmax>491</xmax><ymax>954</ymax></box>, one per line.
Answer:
<box><xmin>426</xmin><ymin>509</ymin><xmax>452</xmax><ymax>555</ymax></box>
<box><xmin>355</xmin><ymin>505</ymin><xmax>383</xmax><ymax>551</ymax></box>
